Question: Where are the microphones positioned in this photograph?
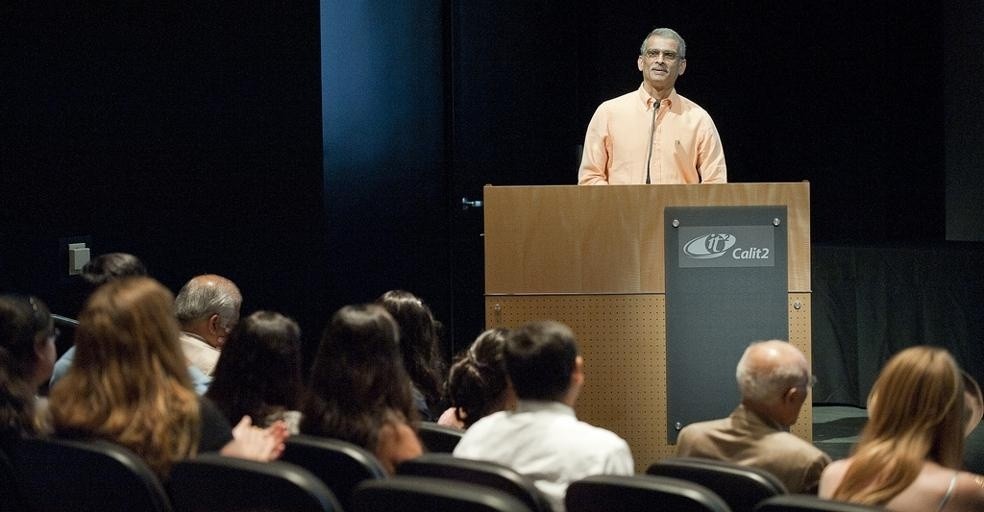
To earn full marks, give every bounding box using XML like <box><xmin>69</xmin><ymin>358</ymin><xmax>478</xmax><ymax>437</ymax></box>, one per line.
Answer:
<box><xmin>646</xmin><ymin>102</ymin><xmax>660</xmax><ymax>185</ymax></box>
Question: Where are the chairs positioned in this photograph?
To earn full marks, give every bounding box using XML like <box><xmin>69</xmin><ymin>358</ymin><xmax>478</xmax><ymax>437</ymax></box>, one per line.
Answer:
<box><xmin>50</xmin><ymin>313</ymin><xmax>82</xmax><ymax>357</ymax></box>
<box><xmin>390</xmin><ymin>457</ymin><xmax>554</xmax><ymax>512</ymax></box>
<box><xmin>754</xmin><ymin>494</ymin><xmax>877</xmax><ymax>512</ymax></box>
<box><xmin>282</xmin><ymin>435</ymin><xmax>383</xmax><ymax>507</ymax></box>
<box><xmin>169</xmin><ymin>455</ymin><xmax>341</xmax><ymax>512</ymax></box>
<box><xmin>647</xmin><ymin>458</ymin><xmax>788</xmax><ymax>511</ymax></box>
<box><xmin>564</xmin><ymin>474</ymin><xmax>728</xmax><ymax>512</ymax></box>
<box><xmin>346</xmin><ymin>476</ymin><xmax>531</xmax><ymax>511</ymax></box>
<box><xmin>415</xmin><ymin>423</ymin><xmax>467</xmax><ymax>456</ymax></box>
<box><xmin>7</xmin><ymin>433</ymin><xmax>172</xmax><ymax>512</ymax></box>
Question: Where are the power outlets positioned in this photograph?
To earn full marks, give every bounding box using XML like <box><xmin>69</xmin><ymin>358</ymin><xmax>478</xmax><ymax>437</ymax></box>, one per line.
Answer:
<box><xmin>69</xmin><ymin>243</ymin><xmax>86</xmax><ymax>275</ymax></box>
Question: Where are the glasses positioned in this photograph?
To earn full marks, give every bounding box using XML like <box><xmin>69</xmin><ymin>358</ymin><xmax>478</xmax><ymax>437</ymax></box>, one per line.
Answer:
<box><xmin>784</xmin><ymin>375</ymin><xmax>817</xmax><ymax>398</ymax></box>
<box><xmin>644</xmin><ymin>47</ymin><xmax>686</xmax><ymax>61</ymax></box>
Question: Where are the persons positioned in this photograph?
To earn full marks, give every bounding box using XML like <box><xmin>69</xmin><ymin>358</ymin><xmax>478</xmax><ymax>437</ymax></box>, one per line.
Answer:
<box><xmin>175</xmin><ymin>275</ymin><xmax>241</xmax><ymax>395</ymax></box>
<box><xmin>381</xmin><ymin>289</ymin><xmax>443</xmax><ymax>419</ymax></box>
<box><xmin>437</xmin><ymin>327</ymin><xmax>515</xmax><ymax>431</ymax></box>
<box><xmin>51</xmin><ymin>254</ymin><xmax>144</xmax><ymax>386</ymax></box>
<box><xmin>676</xmin><ymin>339</ymin><xmax>832</xmax><ymax>498</ymax></box>
<box><xmin>452</xmin><ymin>320</ymin><xmax>634</xmax><ymax>511</ymax></box>
<box><xmin>0</xmin><ymin>296</ymin><xmax>56</xmax><ymax>438</ymax></box>
<box><xmin>51</xmin><ymin>276</ymin><xmax>288</xmax><ymax>464</ymax></box>
<box><xmin>578</xmin><ymin>28</ymin><xmax>728</xmax><ymax>186</ymax></box>
<box><xmin>206</xmin><ymin>310</ymin><xmax>305</xmax><ymax>433</ymax></box>
<box><xmin>819</xmin><ymin>347</ymin><xmax>930</xmax><ymax>512</ymax></box>
<box><xmin>300</xmin><ymin>302</ymin><xmax>424</xmax><ymax>472</ymax></box>
